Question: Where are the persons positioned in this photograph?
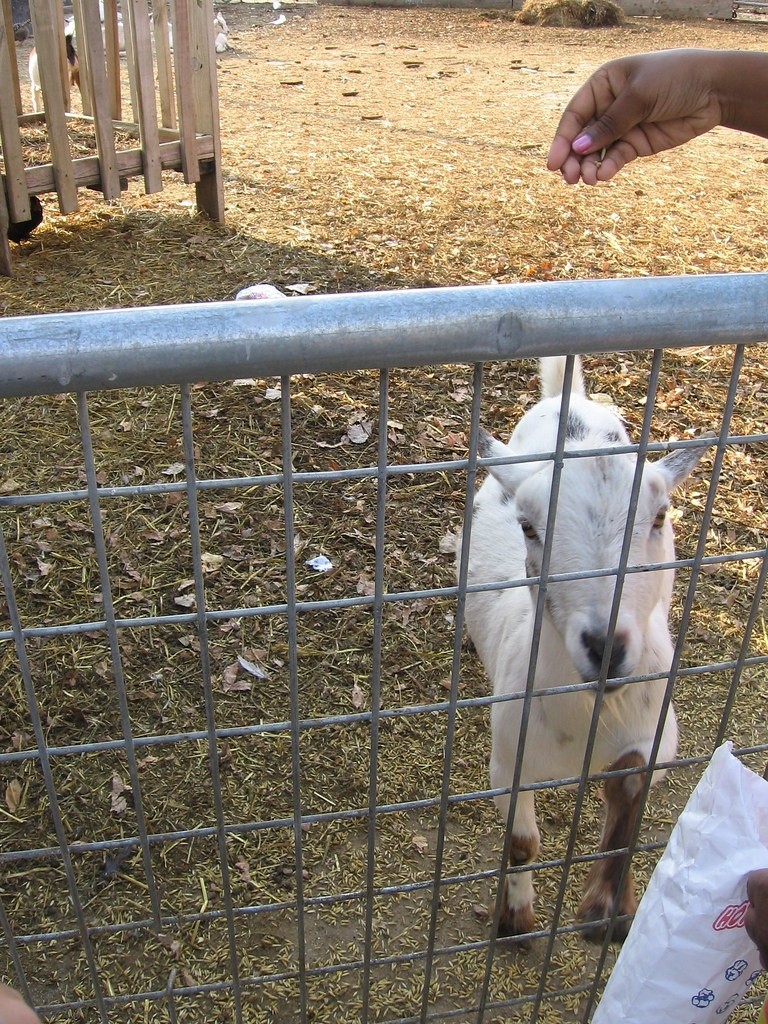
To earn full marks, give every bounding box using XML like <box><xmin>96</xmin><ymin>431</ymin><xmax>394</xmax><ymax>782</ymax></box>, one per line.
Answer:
<box><xmin>544</xmin><ymin>50</ymin><xmax>768</xmax><ymax>973</ymax></box>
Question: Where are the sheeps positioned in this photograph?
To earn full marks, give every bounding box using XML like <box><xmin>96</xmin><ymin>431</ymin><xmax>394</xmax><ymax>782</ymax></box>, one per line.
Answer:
<box><xmin>455</xmin><ymin>358</ymin><xmax>717</xmax><ymax>942</ymax></box>
<box><xmin>29</xmin><ymin>0</ymin><xmax>229</xmax><ymax>113</ymax></box>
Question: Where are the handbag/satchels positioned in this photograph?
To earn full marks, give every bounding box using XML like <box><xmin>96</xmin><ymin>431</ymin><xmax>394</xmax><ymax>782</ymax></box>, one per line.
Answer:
<box><xmin>591</xmin><ymin>741</ymin><xmax>768</xmax><ymax>1024</ymax></box>
